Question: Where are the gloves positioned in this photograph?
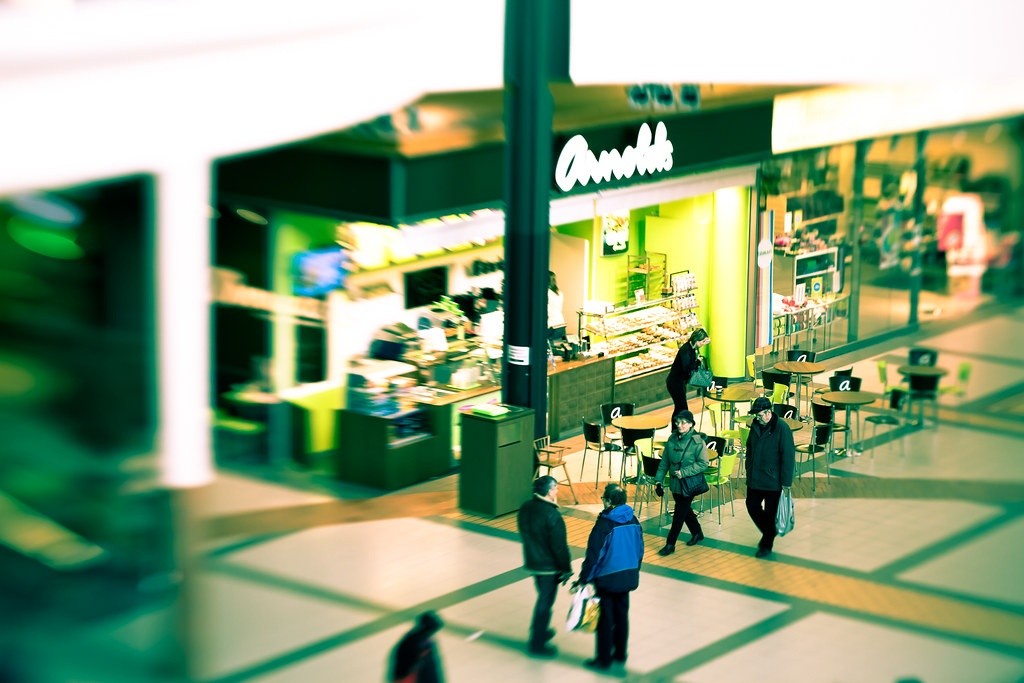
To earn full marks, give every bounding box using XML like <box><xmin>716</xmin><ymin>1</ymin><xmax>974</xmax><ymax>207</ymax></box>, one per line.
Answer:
<box><xmin>655</xmin><ymin>483</ymin><xmax>664</xmax><ymax>498</ymax></box>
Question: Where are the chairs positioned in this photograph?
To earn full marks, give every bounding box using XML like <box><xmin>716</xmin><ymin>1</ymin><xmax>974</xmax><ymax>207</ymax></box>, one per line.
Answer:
<box><xmin>533</xmin><ymin>345</ymin><xmax>973</xmax><ymax>529</ymax></box>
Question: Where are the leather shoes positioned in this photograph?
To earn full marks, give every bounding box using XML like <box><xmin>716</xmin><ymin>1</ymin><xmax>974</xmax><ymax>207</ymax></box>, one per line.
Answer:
<box><xmin>659</xmin><ymin>545</ymin><xmax>675</xmax><ymax>556</ymax></box>
<box><xmin>687</xmin><ymin>532</ymin><xmax>704</xmax><ymax>546</ymax></box>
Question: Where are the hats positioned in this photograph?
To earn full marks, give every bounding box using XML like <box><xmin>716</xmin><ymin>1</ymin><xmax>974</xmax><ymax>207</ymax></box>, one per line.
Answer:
<box><xmin>748</xmin><ymin>397</ymin><xmax>772</xmax><ymax>414</ymax></box>
<box><xmin>673</xmin><ymin>410</ymin><xmax>695</xmax><ymax>428</ymax></box>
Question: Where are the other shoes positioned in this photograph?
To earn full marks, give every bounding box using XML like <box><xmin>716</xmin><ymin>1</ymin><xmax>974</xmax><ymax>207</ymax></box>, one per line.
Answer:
<box><xmin>756</xmin><ymin>537</ymin><xmax>773</xmax><ymax>558</ymax></box>
<box><xmin>528</xmin><ymin>629</ymin><xmax>557</xmax><ymax>655</ymax></box>
<box><xmin>587</xmin><ymin>653</ymin><xmax>627</xmax><ymax>671</ymax></box>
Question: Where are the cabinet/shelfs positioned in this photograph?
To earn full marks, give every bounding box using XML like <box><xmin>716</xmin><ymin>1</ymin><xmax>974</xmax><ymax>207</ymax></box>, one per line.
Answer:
<box><xmin>628</xmin><ymin>251</ymin><xmax>667</xmax><ymax>306</ymax></box>
<box><xmin>775</xmin><ymin>244</ymin><xmax>839</xmax><ymax>301</ymax></box>
<box><xmin>577</xmin><ymin>290</ymin><xmax>702</xmax><ymax>386</ymax></box>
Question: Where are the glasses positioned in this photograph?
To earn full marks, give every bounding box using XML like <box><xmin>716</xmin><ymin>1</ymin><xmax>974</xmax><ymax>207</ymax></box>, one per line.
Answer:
<box><xmin>675</xmin><ymin>420</ymin><xmax>689</xmax><ymax>426</ymax></box>
<box><xmin>755</xmin><ymin>413</ymin><xmax>765</xmax><ymax>418</ymax></box>
<box><xmin>600</xmin><ymin>496</ymin><xmax>606</xmax><ymax>499</ymax></box>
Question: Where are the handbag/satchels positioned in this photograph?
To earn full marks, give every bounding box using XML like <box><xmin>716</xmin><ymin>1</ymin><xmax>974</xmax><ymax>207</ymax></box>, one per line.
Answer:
<box><xmin>681</xmin><ymin>472</ymin><xmax>709</xmax><ymax>497</ymax></box>
<box><xmin>689</xmin><ymin>360</ymin><xmax>712</xmax><ymax>387</ymax></box>
<box><xmin>563</xmin><ymin>582</ymin><xmax>600</xmax><ymax>634</ymax></box>
<box><xmin>776</xmin><ymin>487</ymin><xmax>795</xmax><ymax>538</ymax></box>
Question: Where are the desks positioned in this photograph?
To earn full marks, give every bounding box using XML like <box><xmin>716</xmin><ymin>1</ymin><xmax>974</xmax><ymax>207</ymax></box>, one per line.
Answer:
<box><xmin>773</xmin><ymin>361</ymin><xmax>826</xmax><ymax>423</ymax></box>
<box><xmin>659</xmin><ymin>448</ymin><xmax>718</xmax><ymax>519</ymax></box>
<box><xmin>704</xmin><ymin>387</ymin><xmax>760</xmax><ymax>457</ymax></box>
<box><xmin>821</xmin><ymin>391</ymin><xmax>876</xmax><ymax>458</ymax></box>
<box><xmin>746</xmin><ymin>418</ymin><xmax>804</xmax><ymax>432</ymax></box>
<box><xmin>895</xmin><ymin>365</ymin><xmax>949</xmax><ymax>429</ymax></box>
<box><xmin>610</xmin><ymin>417</ymin><xmax>668</xmax><ymax>486</ymax></box>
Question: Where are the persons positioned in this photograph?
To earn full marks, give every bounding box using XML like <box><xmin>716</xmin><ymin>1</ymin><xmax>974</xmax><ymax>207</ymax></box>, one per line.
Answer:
<box><xmin>418</xmin><ymin>317</ymin><xmax>448</xmax><ymax>354</ymax></box>
<box><xmin>385</xmin><ymin>610</ymin><xmax>444</xmax><ymax>683</ymax></box>
<box><xmin>547</xmin><ymin>271</ymin><xmax>567</xmax><ymax>337</ymax></box>
<box><xmin>654</xmin><ymin>410</ymin><xmax>709</xmax><ymax>556</ymax></box>
<box><xmin>665</xmin><ymin>328</ymin><xmax>710</xmax><ymax>434</ymax></box>
<box><xmin>745</xmin><ymin>397</ymin><xmax>795</xmax><ymax>557</ymax></box>
<box><xmin>570</xmin><ymin>484</ymin><xmax>645</xmax><ymax>670</ymax></box>
<box><xmin>517</xmin><ymin>476</ymin><xmax>574</xmax><ymax>657</ymax></box>
